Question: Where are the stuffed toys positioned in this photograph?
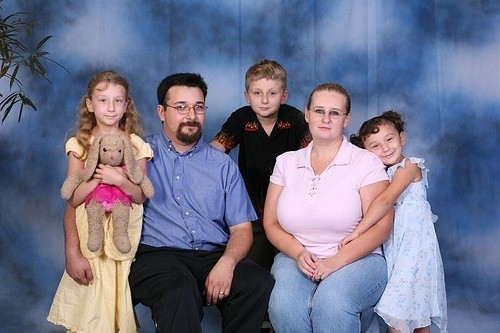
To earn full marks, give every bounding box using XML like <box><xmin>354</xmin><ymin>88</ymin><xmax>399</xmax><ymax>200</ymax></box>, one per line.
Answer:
<box><xmin>60</xmin><ymin>134</ymin><xmax>155</xmax><ymax>254</ymax></box>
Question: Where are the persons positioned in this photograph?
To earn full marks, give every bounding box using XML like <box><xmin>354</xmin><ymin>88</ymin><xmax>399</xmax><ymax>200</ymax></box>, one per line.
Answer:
<box><xmin>263</xmin><ymin>82</ymin><xmax>394</xmax><ymax>333</ymax></box>
<box><xmin>209</xmin><ymin>59</ymin><xmax>313</xmax><ymax>273</ymax></box>
<box><xmin>129</xmin><ymin>73</ymin><xmax>276</xmax><ymax>333</ymax></box>
<box><xmin>46</xmin><ymin>71</ymin><xmax>155</xmax><ymax>333</ymax></box>
<box><xmin>338</xmin><ymin>109</ymin><xmax>448</xmax><ymax>333</ymax></box>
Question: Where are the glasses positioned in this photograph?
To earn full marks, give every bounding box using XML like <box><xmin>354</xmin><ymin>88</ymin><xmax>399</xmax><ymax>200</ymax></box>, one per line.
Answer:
<box><xmin>163</xmin><ymin>102</ymin><xmax>208</xmax><ymax>114</ymax></box>
<box><xmin>307</xmin><ymin>106</ymin><xmax>346</xmax><ymax>119</ymax></box>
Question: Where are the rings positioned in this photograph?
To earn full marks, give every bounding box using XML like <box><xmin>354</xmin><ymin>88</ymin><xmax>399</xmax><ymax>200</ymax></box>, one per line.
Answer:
<box><xmin>219</xmin><ymin>292</ymin><xmax>224</xmax><ymax>295</ymax></box>
<box><xmin>318</xmin><ymin>272</ymin><xmax>322</xmax><ymax>276</ymax></box>
